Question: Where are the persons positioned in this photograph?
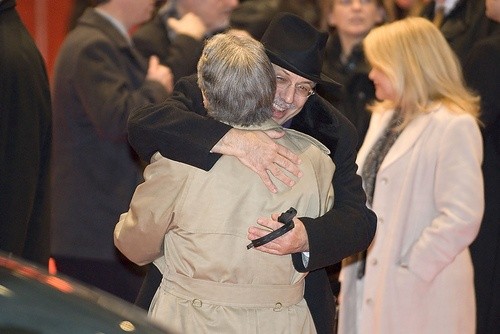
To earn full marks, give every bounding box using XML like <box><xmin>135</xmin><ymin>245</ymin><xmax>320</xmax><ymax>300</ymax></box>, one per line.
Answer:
<box><xmin>0</xmin><ymin>0</ymin><xmax>500</xmax><ymax>334</ymax></box>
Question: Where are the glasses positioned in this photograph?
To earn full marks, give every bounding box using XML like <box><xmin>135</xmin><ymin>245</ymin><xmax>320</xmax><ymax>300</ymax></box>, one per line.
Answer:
<box><xmin>275</xmin><ymin>75</ymin><xmax>315</xmax><ymax>97</ymax></box>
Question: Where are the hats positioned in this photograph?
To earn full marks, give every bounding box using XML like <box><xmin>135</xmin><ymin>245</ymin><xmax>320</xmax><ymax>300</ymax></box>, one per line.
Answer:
<box><xmin>259</xmin><ymin>12</ymin><xmax>344</xmax><ymax>88</ymax></box>
